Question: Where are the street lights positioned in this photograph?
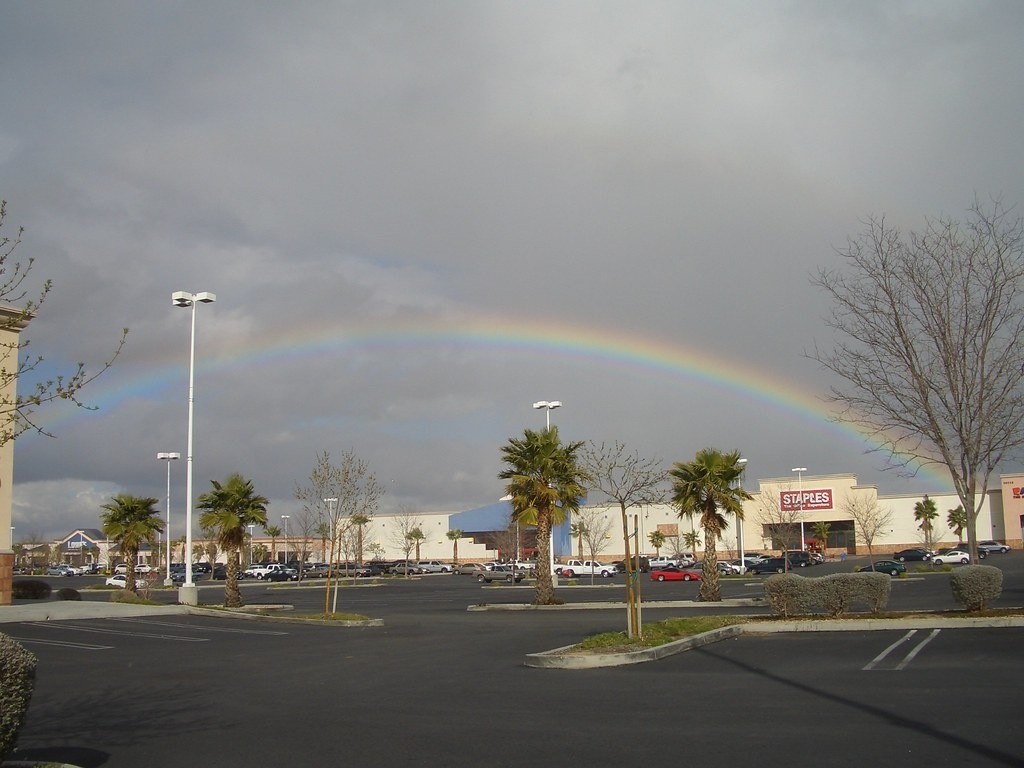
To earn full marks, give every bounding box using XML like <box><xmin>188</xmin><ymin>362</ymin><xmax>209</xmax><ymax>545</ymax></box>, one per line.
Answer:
<box><xmin>156</xmin><ymin>452</ymin><xmax>180</xmax><ymax>587</ymax></box>
<box><xmin>281</xmin><ymin>515</ymin><xmax>290</xmax><ymax>564</ymax></box>
<box><xmin>103</xmin><ymin>523</ymin><xmax>110</xmax><ymax>571</ymax></box>
<box><xmin>171</xmin><ymin>292</ymin><xmax>217</xmax><ymax>605</ymax></box>
<box><xmin>533</xmin><ymin>400</ymin><xmax>563</xmax><ymax>588</ymax></box>
<box><xmin>736</xmin><ymin>459</ymin><xmax>748</xmax><ymax>575</ymax></box>
<box><xmin>323</xmin><ymin>497</ymin><xmax>337</xmax><ymax>554</ymax></box>
<box><xmin>791</xmin><ymin>467</ymin><xmax>807</xmax><ymax>551</ymax></box>
<box><xmin>498</xmin><ymin>495</ymin><xmax>520</xmax><ymax>560</ymax></box>
<box><xmin>247</xmin><ymin>524</ymin><xmax>255</xmax><ymax>565</ymax></box>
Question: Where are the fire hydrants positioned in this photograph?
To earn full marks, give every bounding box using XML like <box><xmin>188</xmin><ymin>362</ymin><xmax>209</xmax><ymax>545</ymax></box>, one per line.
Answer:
<box><xmin>10</xmin><ymin>526</ymin><xmax>16</xmax><ymax>550</ymax></box>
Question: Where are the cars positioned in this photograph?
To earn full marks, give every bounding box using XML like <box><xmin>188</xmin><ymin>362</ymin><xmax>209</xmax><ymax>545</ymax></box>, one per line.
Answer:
<box><xmin>388</xmin><ymin>562</ymin><xmax>430</xmax><ymax>575</ymax></box>
<box><xmin>484</xmin><ymin>562</ymin><xmax>501</xmax><ymax>571</ymax></box>
<box><xmin>170</xmin><ymin>562</ymin><xmax>243</xmax><ymax>582</ymax></box>
<box><xmin>668</xmin><ymin>555</ymin><xmax>691</xmax><ymax>569</ymax></box>
<box><xmin>857</xmin><ymin>560</ymin><xmax>906</xmax><ymax>576</ymax></box>
<box><xmin>651</xmin><ymin>567</ymin><xmax>702</xmax><ymax>582</ymax></box>
<box><xmin>685</xmin><ymin>553</ymin><xmax>772</xmax><ymax>577</ymax></box>
<box><xmin>245</xmin><ymin>561</ymin><xmax>404</xmax><ymax>583</ymax></box>
<box><xmin>105</xmin><ymin>575</ymin><xmax>149</xmax><ymax>590</ymax></box>
<box><xmin>114</xmin><ymin>563</ymin><xmax>141</xmax><ymax>574</ymax></box>
<box><xmin>811</xmin><ymin>553</ymin><xmax>824</xmax><ymax>566</ymax></box>
<box><xmin>12</xmin><ymin>562</ymin><xmax>106</xmax><ymax>577</ymax></box>
<box><xmin>893</xmin><ymin>540</ymin><xmax>1012</xmax><ymax>566</ymax></box>
<box><xmin>452</xmin><ymin>562</ymin><xmax>486</xmax><ymax>575</ymax></box>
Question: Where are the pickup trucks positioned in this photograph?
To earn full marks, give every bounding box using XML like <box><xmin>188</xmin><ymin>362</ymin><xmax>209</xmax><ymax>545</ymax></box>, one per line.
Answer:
<box><xmin>502</xmin><ymin>559</ymin><xmax>536</xmax><ymax>570</ymax></box>
<box><xmin>561</xmin><ymin>560</ymin><xmax>618</xmax><ymax>578</ymax></box>
<box><xmin>554</xmin><ymin>559</ymin><xmax>584</xmax><ymax>575</ymax></box>
<box><xmin>649</xmin><ymin>556</ymin><xmax>679</xmax><ymax>570</ymax></box>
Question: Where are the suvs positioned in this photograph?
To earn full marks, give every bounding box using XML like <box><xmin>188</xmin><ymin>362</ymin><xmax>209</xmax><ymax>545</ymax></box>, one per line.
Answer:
<box><xmin>747</xmin><ymin>557</ymin><xmax>792</xmax><ymax>575</ymax></box>
<box><xmin>782</xmin><ymin>551</ymin><xmax>813</xmax><ymax>568</ymax></box>
<box><xmin>616</xmin><ymin>555</ymin><xmax>650</xmax><ymax>574</ymax></box>
<box><xmin>417</xmin><ymin>560</ymin><xmax>456</xmax><ymax>574</ymax></box>
<box><xmin>136</xmin><ymin>564</ymin><xmax>160</xmax><ymax>574</ymax></box>
<box><xmin>670</xmin><ymin>553</ymin><xmax>698</xmax><ymax>564</ymax></box>
